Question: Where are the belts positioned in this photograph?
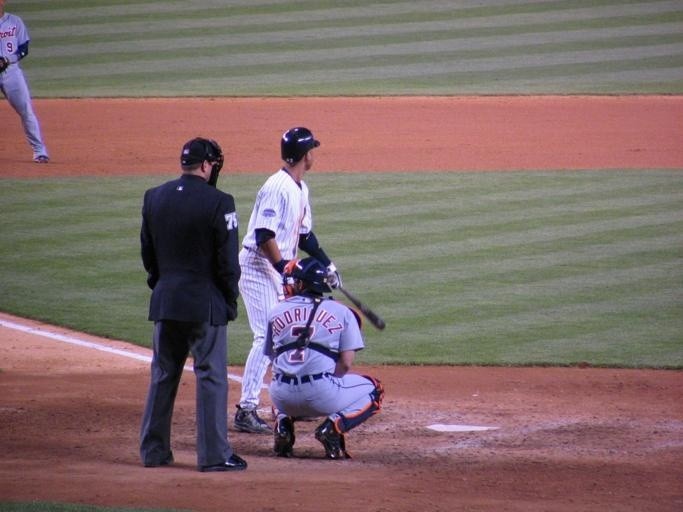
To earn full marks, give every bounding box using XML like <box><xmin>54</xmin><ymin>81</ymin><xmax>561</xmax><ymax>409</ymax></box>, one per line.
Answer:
<box><xmin>274</xmin><ymin>372</ymin><xmax>329</xmax><ymax>385</ymax></box>
<box><xmin>244</xmin><ymin>245</ymin><xmax>267</xmax><ymax>259</ymax></box>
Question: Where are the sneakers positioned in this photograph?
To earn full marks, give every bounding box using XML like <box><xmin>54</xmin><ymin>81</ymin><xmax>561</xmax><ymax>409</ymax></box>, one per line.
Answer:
<box><xmin>233</xmin><ymin>402</ymin><xmax>274</xmax><ymax>436</ymax></box>
<box><xmin>197</xmin><ymin>448</ymin><xmax>248</xmax><ymax>473</ymax></box>
<box><xmin>33</xmin><ymin>148</ymin><xmax>50</xmax><ymax>164</ymax></box>
<box><xmin>313</xmin><ymin>418</ymin><xmax>353</xmax><ymax>462</ymax></box>
<box><xmin>141</xmin><ymin>446</ymin><xmax>174</xmax><ymax>468</ymax></box>
<box><xmin>273</xmin><ymin>414</ymin><xmax>296</xmax><ymax>458</ymax></box>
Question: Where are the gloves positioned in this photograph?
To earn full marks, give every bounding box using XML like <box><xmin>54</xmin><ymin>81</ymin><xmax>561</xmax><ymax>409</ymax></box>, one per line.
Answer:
<box><xmin>326</xmin><ymin>263</ymin><xmax>343</xmax><ymax>289</ymax></box>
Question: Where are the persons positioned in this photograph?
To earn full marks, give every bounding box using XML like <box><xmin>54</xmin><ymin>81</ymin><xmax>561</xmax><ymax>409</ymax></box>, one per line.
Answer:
<box><xmin>0</xmin><ymin>1</ymin><xmax>48</xmax><ymax>162</ymax></box>
<box><xmin>137</xmin><ymin>137</ymin><xmax>247</xmax><ymax>472</ymax></box>
<box><xmin>263</xmin><ymin>257</ymin><xmax>385</xmax><ymax>459</ymax></box>
<box><xmin>233</xmin><ymin>126</ymin><xmax>343</xmax><ymax>434</ymax></box>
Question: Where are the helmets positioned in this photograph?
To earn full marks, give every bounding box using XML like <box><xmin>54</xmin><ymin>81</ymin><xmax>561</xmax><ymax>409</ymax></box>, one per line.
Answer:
<box><xmin>280</xmin><ymin>260</ymin><xmax>332</xmax><ymax>297</ymax></box>
<box><xmin>279</xmin><ymin>125</ymin><xmax>321</xmax><ymax>167</ymax></box>
<box><xmin>181</xmin><ymin>137</ymin><xmax>225</xmax><ymax>185</ymax></box>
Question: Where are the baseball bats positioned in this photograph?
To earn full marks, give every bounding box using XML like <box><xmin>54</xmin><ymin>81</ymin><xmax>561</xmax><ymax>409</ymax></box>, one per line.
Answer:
<box><xmin>338</xmin><ymin>284</ymin><xmax>385</xmax><ymax>329</ymax></box>
